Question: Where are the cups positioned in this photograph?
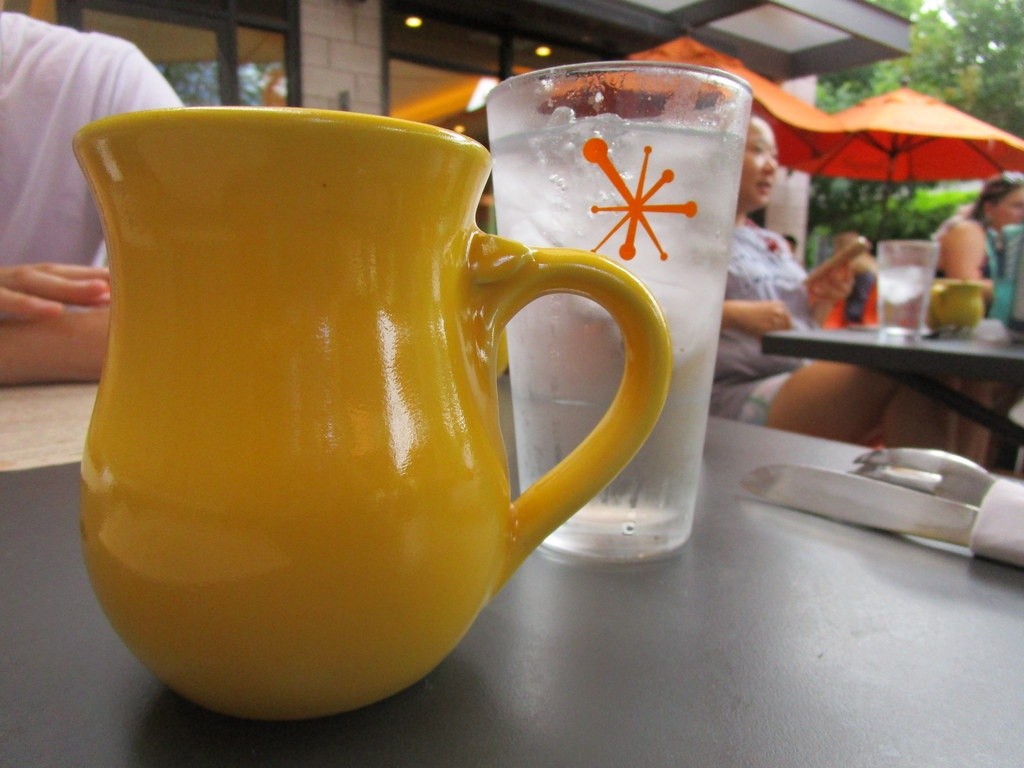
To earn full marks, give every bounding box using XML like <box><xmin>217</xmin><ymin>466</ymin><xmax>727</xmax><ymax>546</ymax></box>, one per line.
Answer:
<box><xmin>876</xmin><ymin>240</ymin><xmax>941</xmax><ymax>345</ymax></box>
<box><xmin>926</xmin><ymin>278</ymin><xmax>985</xmax><ymax>332</ymax></box>
<box><xmin>485</xmin><ymin>61</ymin><xmax>754</xmax><ymax>563</ymax></box>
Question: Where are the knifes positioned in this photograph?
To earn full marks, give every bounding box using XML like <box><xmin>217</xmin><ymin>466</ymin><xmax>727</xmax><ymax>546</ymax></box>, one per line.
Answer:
<box><xmin>851</xmin><ymin>449</ymin><xmax>997</xmax><ymax>506</ymax></box>
<box><xmin>739</xmin><ymin>464</ymin><xmax>978</xmax><ymax>546</ymax></box>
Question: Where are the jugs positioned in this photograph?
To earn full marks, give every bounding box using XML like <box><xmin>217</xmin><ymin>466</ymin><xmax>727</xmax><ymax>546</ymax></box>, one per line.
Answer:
<box><xmin>72</xmin><ymin>106</ymin><xmax>674</xmax><ymax>719</ymax></box>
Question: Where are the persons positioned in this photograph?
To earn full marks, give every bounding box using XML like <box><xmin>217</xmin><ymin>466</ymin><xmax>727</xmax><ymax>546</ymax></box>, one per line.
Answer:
<box><xmin>0</xmin><ymin>11</ymin><xmax>185</xmax><ymax>386</ymax></box>
<box><xmin>804</xmin><ymin>229</ymin><xmax>876</xmax><ymax>328</ymax></box>
<box><xmin>709</xmin><ymin>114</ymin><xmax>990</xmax><ymax>466</ymax></box>
<box><xmin>934</xmin><ymin>171</ymin><xmax>1024</xmax><ymax>323</ymax></box>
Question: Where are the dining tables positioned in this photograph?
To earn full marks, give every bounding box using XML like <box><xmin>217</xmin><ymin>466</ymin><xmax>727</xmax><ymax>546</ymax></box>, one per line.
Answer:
<box><xmin>765</xmin><ymin>324</ymin><xmax>1022</xmax><ymax>471</ymax></box>
<box><xmin>0</xmin><ymin>372</ymin><xmax>1021</xmax><ymax>768</ymax></box>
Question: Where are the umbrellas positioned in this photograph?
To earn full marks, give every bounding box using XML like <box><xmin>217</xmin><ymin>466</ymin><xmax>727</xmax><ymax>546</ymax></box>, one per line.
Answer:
<box><xmin>535</xmin><ymin>36</ymin><xmax>843</xmax><ymax>168</ymax></box>
<box><xmin>786</xmin><ymin>86</ymin><xmax>1024</xmax><ymax>241</ymax></box>
